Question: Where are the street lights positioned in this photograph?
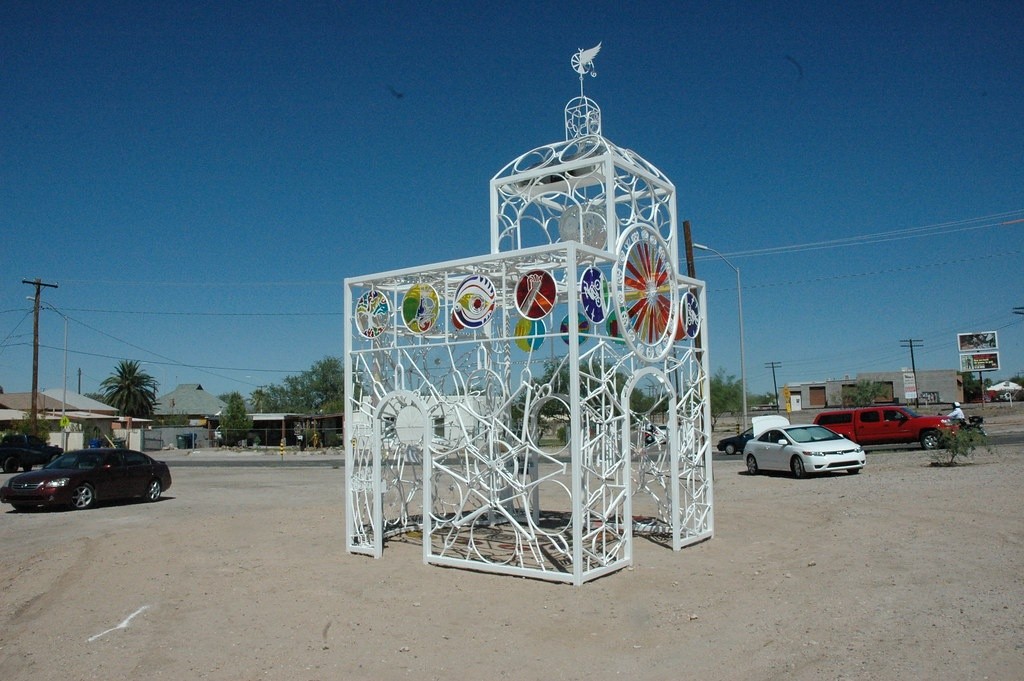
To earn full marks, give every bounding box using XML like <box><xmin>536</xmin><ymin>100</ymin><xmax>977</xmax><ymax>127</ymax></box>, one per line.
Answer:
<box><xmin>693</xmin><ymin>242</ymin><xmax>748</xmax><ymax>432</ymax></box>
<box><xmin>26</xmin><ymin>296</ymin><xmax>69</xmax><ymax>454</ymax></box>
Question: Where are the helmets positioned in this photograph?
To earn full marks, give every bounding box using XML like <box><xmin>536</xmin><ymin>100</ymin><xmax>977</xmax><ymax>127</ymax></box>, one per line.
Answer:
<box><xmin>952</xmin><ymin>402</ymin><xmax>960</xmax><ymax>408</ymax></box>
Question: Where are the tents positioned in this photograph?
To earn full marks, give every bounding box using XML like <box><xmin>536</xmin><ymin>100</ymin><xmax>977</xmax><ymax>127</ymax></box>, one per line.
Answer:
<box><xmin>987</xmin><ymin>380</ymin><xmax>1022</xmax><ymax>401</ymax></box>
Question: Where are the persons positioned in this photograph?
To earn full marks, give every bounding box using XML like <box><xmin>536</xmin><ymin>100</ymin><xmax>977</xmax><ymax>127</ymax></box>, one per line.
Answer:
<box><xmin>947</xmin><ymin>402</ymin><xmax>964</xmax><ymax>418</ymax></box>
<box><xmin>967</xmin><ymin>357</ymin><xmax>972</xmax><ymax>369</ymax></box>
<box><xmin>887</xmin><ymin>411</ymin><xmax>903</xmax><ymax>420</ymax></box>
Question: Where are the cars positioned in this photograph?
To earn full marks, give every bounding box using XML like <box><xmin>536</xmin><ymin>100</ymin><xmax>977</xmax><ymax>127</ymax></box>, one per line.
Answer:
<box><xmin>716</xmin><ymin>426</ymin><xmax>755</xmax><ymax>455</ymax></box>
<box><xmin>741</xmin><ymin>415</ymin><xmax>866</xmax><ymax>478</ymax></box>
<box><xmin>646</xmin><ymin>425</ymin><xmax>669</xmax><ymax>444</ymax></box>
<box><xmin>0</xmin><ymin>446</ymin><xmax>169</xmax><ymax>510</ymax></box>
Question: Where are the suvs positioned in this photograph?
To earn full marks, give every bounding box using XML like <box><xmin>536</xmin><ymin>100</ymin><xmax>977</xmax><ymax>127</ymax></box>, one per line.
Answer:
<box><xmin>0</xmin><ymin>434</ymin><xmax>65</xmax><ymax>473</ymax></box>
<box><xmin>214</xmin><ymin>426</ymin><xmax>225</xmax><ymax>446</ymax></box>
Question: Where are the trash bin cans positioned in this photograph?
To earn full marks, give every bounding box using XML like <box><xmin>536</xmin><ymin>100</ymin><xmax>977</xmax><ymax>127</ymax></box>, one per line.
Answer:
<box><xmin>87</xmin><ymin>439</ymin><xmax>102</xmax><ymax>448</ymax></box>
<box><xmin>112</xmin><ymin>439</ymin><xmax>126</xmax><ymax>449</ymax></box>
<box><xmin>176</xmin><ymin>433</ymin><xmax>197</xmax><ymax>449</ymax></box>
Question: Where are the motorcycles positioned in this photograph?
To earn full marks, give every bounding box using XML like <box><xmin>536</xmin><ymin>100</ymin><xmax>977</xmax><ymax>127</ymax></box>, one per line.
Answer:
<box><xmin>936</xmin><ymin>412</ymin><xmax>988</xmax><ymax>438</ymax></box>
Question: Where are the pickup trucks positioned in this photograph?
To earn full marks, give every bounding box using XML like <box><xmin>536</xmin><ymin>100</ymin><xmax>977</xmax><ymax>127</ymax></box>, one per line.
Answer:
<box><xmin>812</xmin><ymin>406</ymin><xmax>958</xmax><ymax>451</ymax></box>
